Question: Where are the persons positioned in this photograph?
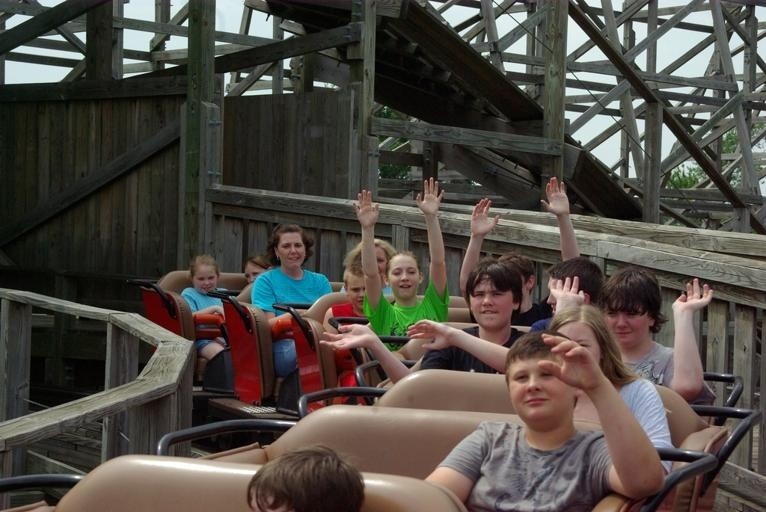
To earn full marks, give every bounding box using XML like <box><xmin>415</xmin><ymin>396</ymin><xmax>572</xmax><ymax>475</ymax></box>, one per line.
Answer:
<box><xmin>180</xmin><ymin>254</ymin><xmax>233</xmax><ymax>391</ymax></box>
<box><xmin>249</xmin><ymin>221</ymin><xmax>334</xmax><ymax>379</ymax></box>
<box><xmin>547</xmin><ymin>304</ymin><xmax>677</xmax><ymax>477</ymax></box>
<box><xmin>549</xmin><ymin>266</ymin><xmax>714</xmax><ymax>401</ymax></box>
<box><xmin>424</xmin><ymin>329</ymin><xmax>665</xmax><ymax>511</ymax></box>
<box><xmin>323</xmin><ymin>263</ymin><xmax>373</xmax><ymax>406</ymax></box>
<box><xmin>233</xmin><ymin>253</ymin><xmax>276</xmax><ymax>304</ymax></box>
<box><xmin>531</xmin><ymin>255</ymin><xmax>606</xmax><ymax>334</ymax></box>
<box><xmin>352</xmin><ymin>175</ymin><xmax>451</xmax><ymax>360</ymax></box>
<box><xmin>459</xmin><ymin>175</ymin><xmax>582</xmax><ymax>327</ymax></box>
<box><xmin>246</xmin><ymin>443</ymin><xmax>365</xmax><ymax>511</ymax></box>
<box><xmin>341</xmin><ymin>236</ymin><xmax>397</xmax><ymax>295</ymax></box>
<box><xmin>318</xmin><ymin>254</ymin><xmax>527</xmax><ymax>385</ymax></box>
